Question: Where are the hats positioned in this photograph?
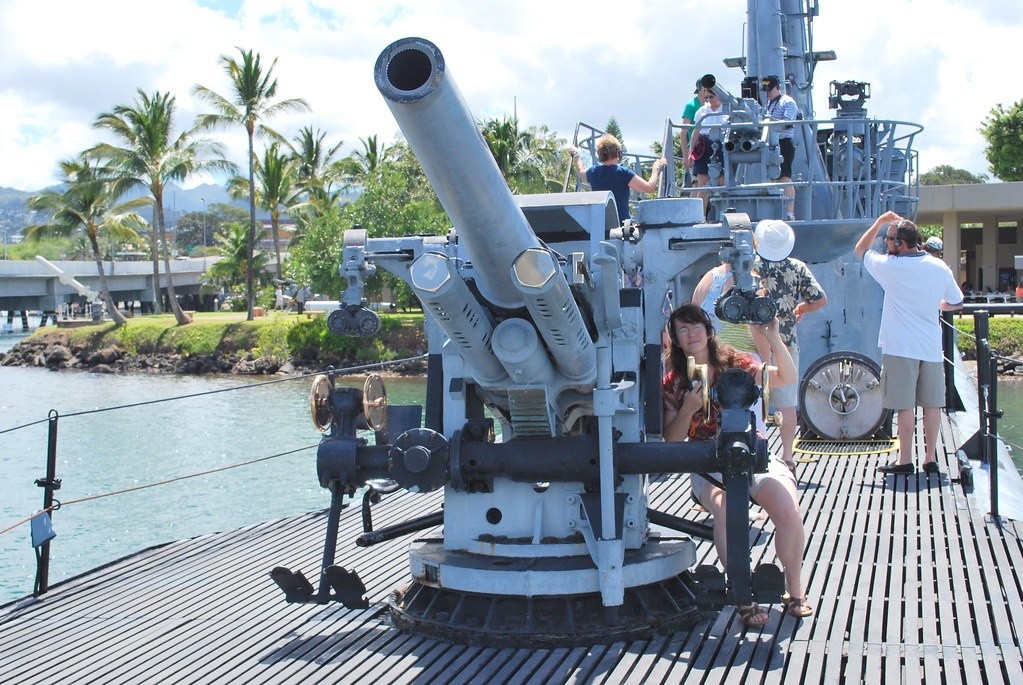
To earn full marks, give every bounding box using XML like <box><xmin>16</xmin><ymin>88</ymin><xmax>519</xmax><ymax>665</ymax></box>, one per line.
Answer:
<box><xmin>753</xmin><ymin>219</ymin><xmax>795</xmax><ymax>261</ymax></box>
<box><xmin>760</xmin><ymin>75</ymin><xmax>779</xmax><ymax>91</ymax></box>
<box><xmin>690</xmin><ymin>137</ymin><xmax>706</xmax><ymax>160</ymax></box>
<box><xmin>693</xmin><ymin>79</ymin><xmax>704</xmax><ymax>94</ymax></box>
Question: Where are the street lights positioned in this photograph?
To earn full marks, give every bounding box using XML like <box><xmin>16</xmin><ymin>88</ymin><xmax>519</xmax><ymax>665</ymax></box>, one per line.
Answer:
<box><xmin>200</xmin><ymin>197</ymin><xmax>206</xmax><ymax>248</ymax></box>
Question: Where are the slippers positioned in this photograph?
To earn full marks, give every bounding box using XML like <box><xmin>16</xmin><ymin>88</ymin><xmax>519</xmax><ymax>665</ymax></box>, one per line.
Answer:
<box><xmin>739</xmin><ymin>604</ymin><xmax>770</xmax><ymax>625</ymax></box>
<box><xmin>781</xmin><ymin>594</ymin><xmax>812</xmax><ymax>617</ymax></box>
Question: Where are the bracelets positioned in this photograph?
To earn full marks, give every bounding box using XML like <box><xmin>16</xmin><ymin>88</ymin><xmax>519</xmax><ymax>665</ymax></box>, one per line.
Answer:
<box><xmin>574</xmin><ymin>154</ymin><xmax>580</xmax><ymax>158</ymax></box>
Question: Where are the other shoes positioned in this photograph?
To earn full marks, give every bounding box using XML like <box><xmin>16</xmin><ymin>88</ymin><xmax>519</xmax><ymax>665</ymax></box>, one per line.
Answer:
<box><xmin>878</xmin><ymin>460</ymin><xmax>914</xmax><ymax>474</ymax></box>
<box><xmin>783</xmin><ymin>460</ymin><xmax>795</xmax><ymax>476</ymax></box>
<box><xmin>783</xmin><ymin>213</ymin><xmax>795</xmax><ymax>221</ymax></box>
<box><xmin>922</xmin><ymin>460</ymin><xmax>938</xmax><ymax>472</ymax></box>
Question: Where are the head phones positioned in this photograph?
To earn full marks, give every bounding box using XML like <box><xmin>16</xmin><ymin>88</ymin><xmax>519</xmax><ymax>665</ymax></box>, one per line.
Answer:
<box><xmin>768</xmin><ymin>74</ymin><xmax>781</xmax><ymax>91</ymax></box>
<box><xmin>894</xmin><ymin>220</ymin><xmax>902</xmax><ymax>247</ymax></box>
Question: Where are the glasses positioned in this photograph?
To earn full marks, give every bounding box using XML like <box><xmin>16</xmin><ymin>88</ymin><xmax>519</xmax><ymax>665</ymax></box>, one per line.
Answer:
<box><xmin>677</xmin><ymin>324</ymin><xmax>702</xmax><ymax>335</ymax></box>
<box><xmin>886</xmin><ymin>236</ymin><xmax>905</xmax><ymax>241</ymax></box>
<box><xmin>704</xmin><ymin>95</ymin><xmax>714</xmax><ymax>99</ymax></box>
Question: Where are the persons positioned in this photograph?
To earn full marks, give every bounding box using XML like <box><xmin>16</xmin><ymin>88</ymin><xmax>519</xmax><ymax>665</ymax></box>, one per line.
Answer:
<box><xmin>570</xmin><ymin>133</ymin><xmax>668</xmax><ymax>226</ymax></box>
<box><xmin>692</xmin><ymin>242</ymin><xmax>771</xmax><ymax>433</ymax></box>
<box><xmin>961</xmin><ymin>282</ymin><xmax>975</xmax><ymax>301</ymax></box>
<box><xmin>1016</xmin><ymin>281</ymin><xmax>1023</xmax><ymax>303</ymax></box>
<box><xmin>761</xmin><ymin>74</ymin><xmax>798</xmax><ymax>221</ymax></box>
<box><xmin>680</xmin><ymin>78</ymin><xmax>725</xmax><ymax>214</ymax></box>
<box><xmin>274</xmin><ymin>285</ymin><xmax>284</xmax><ymax>312</ymax></box>
<box><xmin>986</xmin><ymin>287</ymin><xmax>999</xmax><ymax>295</ymax></box>
<box><xmin>854</xmin><ymin>210</ymin><xmax>964</xmax><ymax>474</ymax></box>
<box><xmin>751</xmin><ymin>220</ymin><xmax>828</xmax><ymax>477</ymax></box>
<box><xmin>297</xmin><ymin>289</ymin><xmax>304</xmax><ymax>314</ymax></box>
<box><xmin>923</xmin><ymin>237</ymin><xmax>942</xmax><ymax>258</ymax></box>
<box><xmin>663</xmin><ymin>304</ymin><xmax>813</xmax><ymax>626</ymax></box>
